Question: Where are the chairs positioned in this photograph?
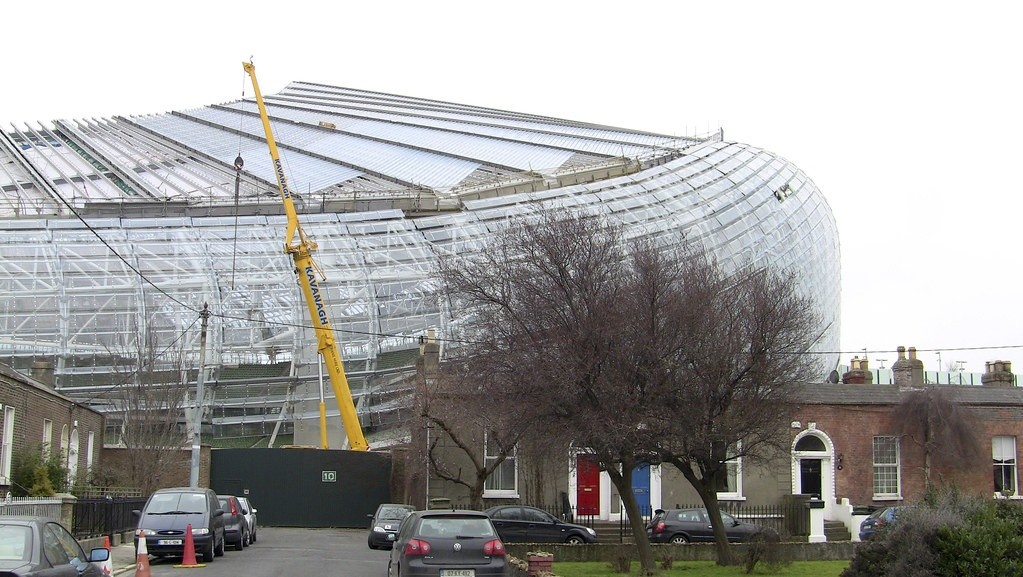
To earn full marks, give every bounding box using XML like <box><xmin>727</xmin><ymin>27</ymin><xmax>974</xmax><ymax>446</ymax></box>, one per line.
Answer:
<box><xmin>222</xmin><ymin>503</ymin><xmax>227</xmax><ymax>512</ymax></box>
<box><xmin>421</xmin><ymin>525</ymin><xmax>433</xmax><ymax>535</ymax></box>
<box><xmin>461</xmin><ymin>525</ymin><xmax>477</xmax><ymax>535</ymax></box>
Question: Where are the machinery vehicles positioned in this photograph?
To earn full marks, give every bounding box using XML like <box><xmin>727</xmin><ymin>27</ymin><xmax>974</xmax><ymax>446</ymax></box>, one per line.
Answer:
<box><xmin>232</xmin><ymin>59</ymin><xmax>372</xmax><ymax>451</ymax></box>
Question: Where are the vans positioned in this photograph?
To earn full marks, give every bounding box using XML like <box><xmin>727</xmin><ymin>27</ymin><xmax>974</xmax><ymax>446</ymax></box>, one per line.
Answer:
<box><xmin>130</xmin><ymin>486</ymin><xmax>226</xmax><ymax>563</ymax></box>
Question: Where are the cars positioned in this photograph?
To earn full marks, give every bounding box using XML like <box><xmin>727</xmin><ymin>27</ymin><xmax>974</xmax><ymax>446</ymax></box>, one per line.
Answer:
<box><xmin>217</xmin><ymin>494</ymin><xmax>252</xmax><ymax>551</ymax></box>
<box><xmin>220</xmin><ymin>496</ymin><xmax>259</xmax><ymax>545</ymax></box>
<box><xmin>859</xmin><ymin>504</ymin><xmax>920</xmax><ymax>542</ymax></box>
<box><xmin>476</xmin><ymin>504</ymin><xmax>598</xmax><ymax>545</ymax></box>
<box><xmin>0</xmin><ymin>515</ymin><xmax>110</xmax><ymax>577</ymax></box>
<box><xmin>388</xmin><ymin>509</ymin><xmax>514</xmax><ymax>577</ymax></box>
<box><xmin>366</xmin><ymin>503</ymin><xmax>419</xmax><ymax>549</ymax></box>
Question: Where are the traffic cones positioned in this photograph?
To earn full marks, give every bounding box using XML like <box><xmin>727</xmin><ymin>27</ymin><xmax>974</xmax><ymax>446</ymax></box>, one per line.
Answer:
<box><xmin>99</xmin><ymin>535</ymin><xmax>114</xmax><ymax>577</ymax></box>
<box><xmin>172</xmin><ymin>523</ymin><xmax>207</xmax><ymax>568</ymax></box>
<box><xmin>134</xmin><ymin>529</ymin><xmax>151</xmax><ymax>577</ymax></box>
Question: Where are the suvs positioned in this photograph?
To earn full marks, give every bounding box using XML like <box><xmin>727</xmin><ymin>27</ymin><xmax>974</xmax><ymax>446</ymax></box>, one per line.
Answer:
<box><xmin>645</xmin><ymin>508</ymin><xmax>782</xmax><ymax>545</ymax></box>
